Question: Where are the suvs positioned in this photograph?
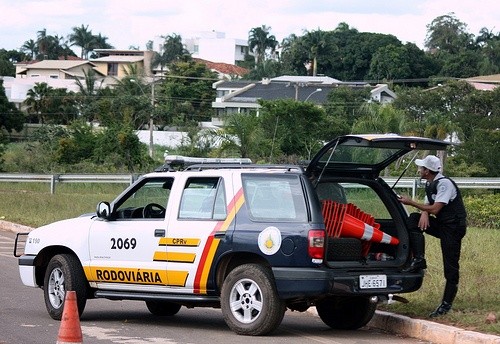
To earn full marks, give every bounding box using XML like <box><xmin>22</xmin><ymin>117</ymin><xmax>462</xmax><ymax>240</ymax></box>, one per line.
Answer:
<box><xmin>13</xmin><ymin>132</ymin><xmax>459</xmax><ymax>338</ymax></box>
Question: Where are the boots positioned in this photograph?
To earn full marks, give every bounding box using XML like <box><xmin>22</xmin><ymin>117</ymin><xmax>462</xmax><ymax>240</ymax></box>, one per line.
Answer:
<box><xmin>430</xmin><ymin>285</ymin><xmax>458</xmax><ymax>318</ymax></box>
<box><xmin>400</xmin><ymin>231</ymin><xmax>427</xmax><ymax>272</ymax></box>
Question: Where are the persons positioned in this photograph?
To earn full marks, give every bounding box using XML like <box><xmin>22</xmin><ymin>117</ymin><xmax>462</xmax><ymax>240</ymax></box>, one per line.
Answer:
<box><xmin>396</xmin><ymin>155</ymin><xmax>466</xmax><ymax>320</ymax></box>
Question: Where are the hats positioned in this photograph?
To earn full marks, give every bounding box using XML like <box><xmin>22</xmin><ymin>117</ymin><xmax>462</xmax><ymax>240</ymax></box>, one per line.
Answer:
<box><xmin>416</xmin><ymin>154</ymin><xmax>441</xmax><ymax>172</ymax></box>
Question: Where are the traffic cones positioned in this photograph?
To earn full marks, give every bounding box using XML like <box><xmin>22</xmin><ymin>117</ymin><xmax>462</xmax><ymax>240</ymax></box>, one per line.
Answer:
<box><xmin>320</xmin><ymin>199</ymin><xmax>399</xmax><ymax>245</ymax></box>
<box><xmin>54</xmin><ymin>289</ymin><xmax>86</xmax><ymax>344</ymax></box>
